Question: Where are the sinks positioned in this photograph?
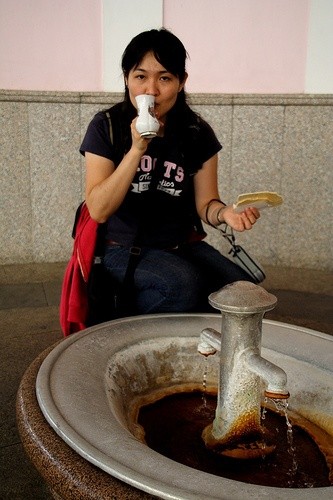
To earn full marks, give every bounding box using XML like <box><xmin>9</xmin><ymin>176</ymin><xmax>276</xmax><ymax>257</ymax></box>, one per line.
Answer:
<box><xmin>16</xmin><ymin>313</ymin><xmax>333</xmax><ymax>500</ymax></box>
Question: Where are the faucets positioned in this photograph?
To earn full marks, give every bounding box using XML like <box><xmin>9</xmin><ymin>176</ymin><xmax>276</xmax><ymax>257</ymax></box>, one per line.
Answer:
<box><xmin>196</xmin><ymin>328</ymin><xmax>224</xmax><ymax>356</ymax></box>
<box><xmin>247</xmin><ymin>351</ymin><xmax>291</xmax><ymax>401</ymax></box>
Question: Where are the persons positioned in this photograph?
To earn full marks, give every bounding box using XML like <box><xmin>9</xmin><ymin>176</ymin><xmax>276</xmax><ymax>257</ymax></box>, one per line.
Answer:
<box><xmin>78</xmin><ymin>27</ymin><xmax>262</xmax><ymax>314</ymax></box>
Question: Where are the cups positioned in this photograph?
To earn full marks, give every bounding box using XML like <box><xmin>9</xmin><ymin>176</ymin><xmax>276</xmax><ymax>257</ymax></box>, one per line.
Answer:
<box><xmin>135</xmin><ymin>94</ymin><xmax>160</xmax><ymax>139</ymax></box>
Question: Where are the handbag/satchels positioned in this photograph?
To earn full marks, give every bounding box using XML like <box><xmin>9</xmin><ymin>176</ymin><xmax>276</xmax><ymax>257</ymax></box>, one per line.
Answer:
<box><xmin>206</xmin><ymin>199</ymin><xmax>266</xmax><ymax>283</ymax></box>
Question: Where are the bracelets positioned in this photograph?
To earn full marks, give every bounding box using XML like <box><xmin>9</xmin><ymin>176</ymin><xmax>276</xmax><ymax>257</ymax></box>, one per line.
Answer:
<box><xmin>216</xmin><ymin>205</ymin><xmax>227</xmax><ymax>225</ymax></box>
